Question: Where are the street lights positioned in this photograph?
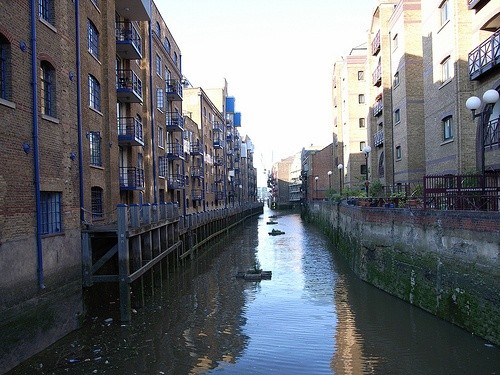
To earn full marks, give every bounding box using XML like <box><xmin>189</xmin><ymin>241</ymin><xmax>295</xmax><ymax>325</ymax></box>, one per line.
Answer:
<box><xmin>314</xmin><ymin>177</ymin><xmax>319</xmax><ymax>198</ymax></box>
<box><xmin>337</xmin><ymin>163</ymin><xmax>343</xmax><ymax>195</ymax></box>
<box><xmin>363</xmin><ymin>146</ymin><xmax>371</xmax><ymax>197</ymax></box>
<box><xmin>327</xmin><ymin>171</ymin><xmax>333</xmax><ymax>194</ymax></box>
<box><xmin>464</xmin><ymin>88</ymin><xmax>500</xmax><ymax>212</ymax></box>
<box><xmin>158</xmin><ymin>175</ymin><xmax>168</xmax><ymax>205</ymax></box>
<box><xmin>233</xmin><ymin>184</ymin><xmax>242</xmax><ymax>207</ymax></box>
